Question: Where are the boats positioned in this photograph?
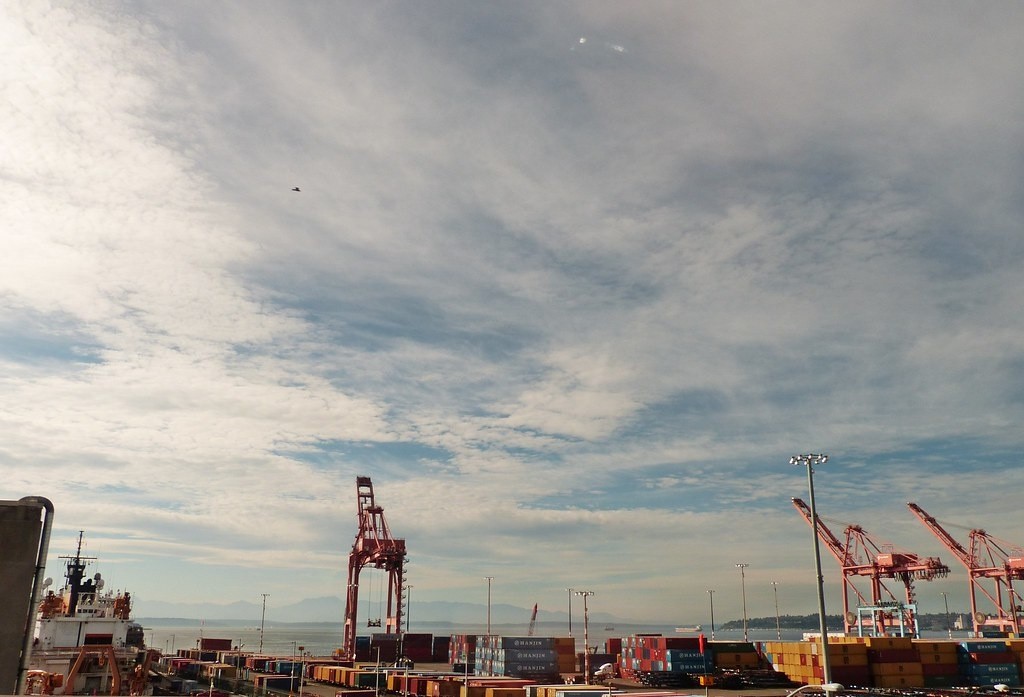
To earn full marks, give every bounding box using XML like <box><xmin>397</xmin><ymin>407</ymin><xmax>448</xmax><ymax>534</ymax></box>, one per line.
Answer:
<box><xmin>675</xmin><ymin>624</ymin><xmax>702</xmax><ymax>634</ymax></box>
<box><xmin>34</xmin><ymin>531</ymin><xmax>143</xmax><ymax>652</ymax></box>
<box><xmin>605</xmin><ymin>626</ymin><xmax>614</xmax><ymax>631</ymax></box>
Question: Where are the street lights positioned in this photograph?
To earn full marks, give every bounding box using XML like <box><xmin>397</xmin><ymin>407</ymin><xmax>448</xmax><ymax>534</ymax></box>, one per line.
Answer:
<box><xmin>940</xmin><ymin>592</ymin><xmax>952</xmax><ymax>641</ymax></box>
<box><xmin>574</xmin><ymin>590</ymin><xmax>595</xmax><ymax>684</ymax></box>
<box><xmin>791</xmin><ymin>454</ymin><xmax>832</xmax><ymax>697</ymax></box>
<box><xmin>735</xmin><ymin>563</ymin><xmax>750</xmax><ymax>643</ymax></box>
<box><xmin>484</xmin><ymin>576</ymin><xmax>494</xmax><ymax>635</ymax></box>
<box><xmin>565</xmin><ymin>588</ymin><xmax>575</xmax><ymax>638</ymax></box>
<box><xmin>707</xmin><ymin>590</ymin><xmax>716</xmax><ymax>642</ymax></box>
<box><xmin>770</xmin><ymin>582</ymin><xmax>781</xmax><ymax>640</ymax></box>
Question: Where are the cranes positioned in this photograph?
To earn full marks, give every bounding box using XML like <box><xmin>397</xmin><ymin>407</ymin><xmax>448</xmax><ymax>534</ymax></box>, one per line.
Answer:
<box><xmin>338</xmin><ymin>473</ymin><xmax>408</xmax><ymax>663</ymax></box>
<box><xmin>905</xmin><ymin>500</ymin><xmax>1024</xmax><ymax>641</ymax></box>
<box><xmin>789</xmin><ymin>498</ymin><xmax>950</xmax><ymax>638</ymax></box>
